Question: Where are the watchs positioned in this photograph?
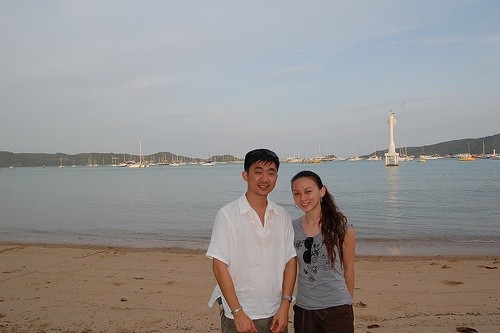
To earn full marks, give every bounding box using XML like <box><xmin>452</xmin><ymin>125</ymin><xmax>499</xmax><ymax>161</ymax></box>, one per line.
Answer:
<box><xmin>281</xmin><ymin>294</ymin><xmax>293</xmax><ymax>303</ymax></box>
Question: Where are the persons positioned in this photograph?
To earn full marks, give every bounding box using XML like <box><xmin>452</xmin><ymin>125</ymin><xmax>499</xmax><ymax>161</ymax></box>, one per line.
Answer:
<box><xmin>290</xmin><ymin>170</ymin><xmax>357</xmax><ymax>333</ymax></box>
<box><xmin>205</xmin><ymin>148</ymin><xmax>298</xmax><ymax>333</ymax></box>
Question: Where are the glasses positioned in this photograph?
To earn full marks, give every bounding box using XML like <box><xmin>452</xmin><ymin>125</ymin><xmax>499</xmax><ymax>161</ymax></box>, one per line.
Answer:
<box><xmin>303</xmin><ymin>237</ymin><xmax>314</xmax><ymax>264</ymax></box>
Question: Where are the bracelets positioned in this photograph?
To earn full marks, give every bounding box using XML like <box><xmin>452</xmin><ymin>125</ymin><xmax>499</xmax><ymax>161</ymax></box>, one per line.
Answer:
<box><xmin>231</xmin><ymin>305</ymin><xmax>242</xmax><ymax>314</ymax></box>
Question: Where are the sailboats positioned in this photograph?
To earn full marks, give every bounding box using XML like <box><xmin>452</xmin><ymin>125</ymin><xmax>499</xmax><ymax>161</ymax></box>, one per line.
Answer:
<box><xmin>458</xmin><ymin>141</ymin><xmax>500</xmax><ymax>160</ymax></box>
<box><xmin>110</xmin><ymin>140</ymin><xmax>216</xmax><ymax>168</ymax></box>
<box><xmin>287</xmin><ymin>144</ymin><xmax>320</xmax><ymax>164</ymax></box>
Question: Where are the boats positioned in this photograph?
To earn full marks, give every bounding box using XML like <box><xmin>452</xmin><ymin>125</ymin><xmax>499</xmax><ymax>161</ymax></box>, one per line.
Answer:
<box><xmin>420</xmin><ymin>155</ymin><xmax>443</xmax><ymax>159</ymax></box>
